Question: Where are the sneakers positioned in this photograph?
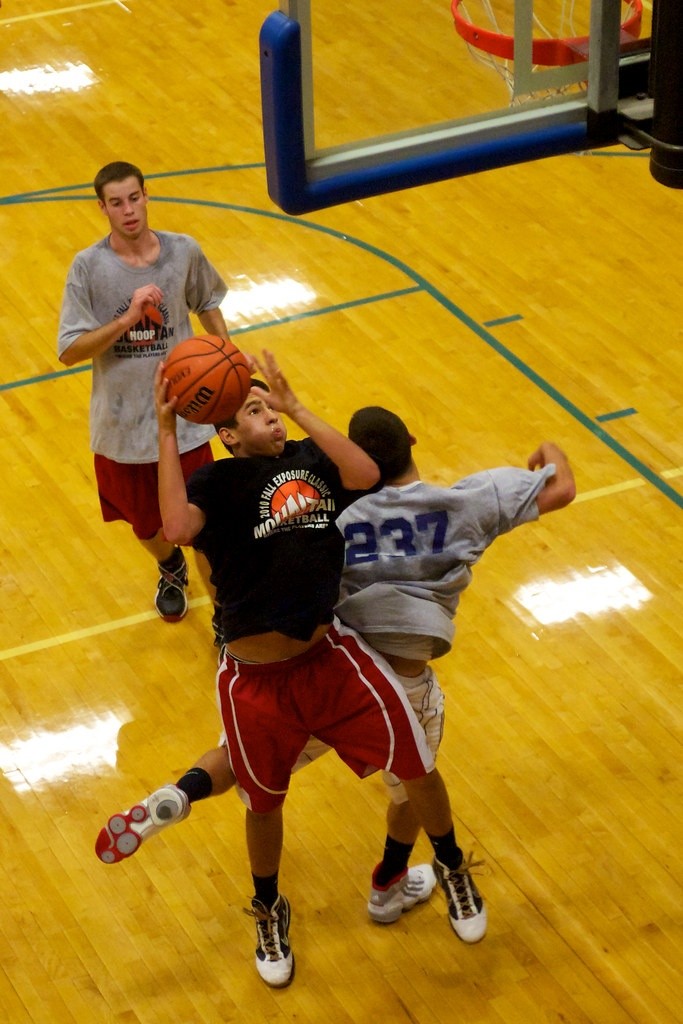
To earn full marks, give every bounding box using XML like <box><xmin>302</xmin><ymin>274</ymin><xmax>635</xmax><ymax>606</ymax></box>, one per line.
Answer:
<box><xmin>432</xmin><ymin>846</ymin><xmax>488</xmax><ymax>944</ymax></box>
<box><xmin>94</xmin><ymin>784</ymin><xmax>192</xmax><ymax>864</ymax></box>
<box><xmin>366</xmin><ymin>859</ymin><xmax>437</xmax><ymax>922</ymax></box>
<box><xmin>244</xmin><ymin>891</ymin><xmax>294</xmax><ymax>988</ymax></box>
<box><xmin>154</xmin><ymin>545</ymin><xmax>188</xmax><ymax>621</ymax></box>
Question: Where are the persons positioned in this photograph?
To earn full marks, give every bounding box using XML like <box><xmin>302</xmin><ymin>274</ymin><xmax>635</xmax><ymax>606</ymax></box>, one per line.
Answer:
<box><xmin>95</xmin><ymin>406</ymin><xmax>576</xmax><ymax>924</ymax></box>
<box><xmin>153</xmin><ymin>349</ymin><xmax>488</xmax><ymax>989</ymax></box>
<box><xmin>58</xmin><ymin>162</ymin><xmax>257</xmax><ymax>623</ymax></box>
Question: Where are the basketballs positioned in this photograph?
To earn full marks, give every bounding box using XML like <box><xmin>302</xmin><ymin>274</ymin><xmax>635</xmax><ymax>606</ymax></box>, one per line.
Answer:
<box><xmin>162</xmin><ymin>335</ymin><xmax>253</xmax><ymax>423</ymax></box>
